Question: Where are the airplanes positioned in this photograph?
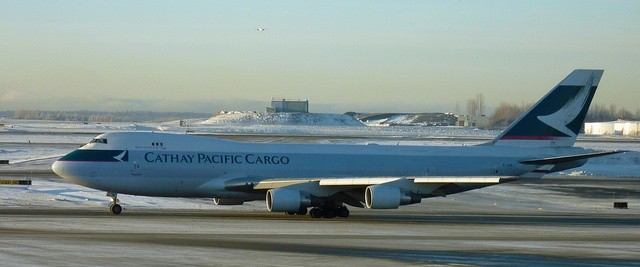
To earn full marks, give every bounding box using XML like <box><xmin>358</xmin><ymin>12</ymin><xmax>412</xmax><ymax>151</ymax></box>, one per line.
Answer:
<box><xmin>52</xmin><ymin>68</ymin><xmax>630</xmax><ymax>219</ymax></box>
